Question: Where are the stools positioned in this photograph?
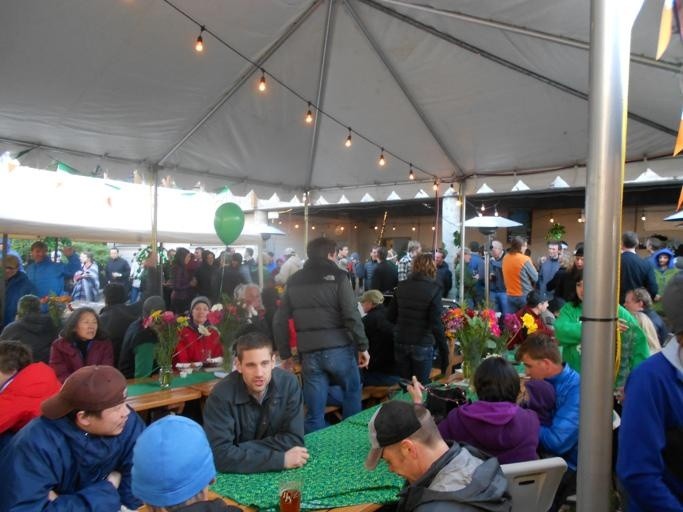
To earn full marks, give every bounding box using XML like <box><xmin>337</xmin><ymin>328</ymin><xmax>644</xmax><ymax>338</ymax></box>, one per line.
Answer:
<box><xmin>322</xmin><ymin>401</ymin><xmax>342</xmax><ymax>422</ymax></box>
<box><xmin>361</xmin><ymin>389</ymin><xmax>369</xmax><ymax>399</ymax></box>
<box><xmin>429</xmin><ymin>367</ymin><xmax>441</xmax><ymax>382</ymax></box>
<box><xmin>366</xmin><ymin>382</ymin><xmax>402</xmax><ymax>399</ymax></box>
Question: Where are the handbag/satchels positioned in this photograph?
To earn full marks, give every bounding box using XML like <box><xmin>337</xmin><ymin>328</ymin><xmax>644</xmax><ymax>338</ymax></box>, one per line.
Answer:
<box><xmin>424</xmin><ymin>387</ymin><xmax>471</xmax><ymax>424</ymax></box>
<box><xmin>171</xmin><ymin>290</ymin><xmax>187</xmax><ymax>300</ymax></box>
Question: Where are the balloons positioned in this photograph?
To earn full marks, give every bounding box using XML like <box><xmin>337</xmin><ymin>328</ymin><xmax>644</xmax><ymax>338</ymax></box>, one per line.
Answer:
<box><xmin>213</xmin><ymin>201</ymin><xmax>245</xmax><ymax>247</ymax></box>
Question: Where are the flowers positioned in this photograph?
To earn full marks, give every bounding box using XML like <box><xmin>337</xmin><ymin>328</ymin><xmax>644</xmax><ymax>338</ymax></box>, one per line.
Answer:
<box><xmin>39</xmin><ymin>289</ymin><xmax>74</xmax><ymax>328</ymax></box>
<box><xmin>204</xmin><ymin>291</ymin><xmax>265</xmax><ymax>372</ymax></box>
<box><xmin>142</xmin><ymin>309</ymin><xmax>189</xmax><ymax>389</ymax></box>
<box><xmin>440</xmin><ymin>298</ymin><xmax>539</xmax><ymax>403</ymax></box>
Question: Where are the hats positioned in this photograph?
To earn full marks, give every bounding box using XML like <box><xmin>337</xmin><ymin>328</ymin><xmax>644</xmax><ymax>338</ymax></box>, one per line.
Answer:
<box><xmin>191</xmin><ymin>296</ymin><xmax>211</xmax><ymax>312</ymax></box>
<box><xmin>527</xmin><ymin>290</ymin><xmax>553</xmax><ymax>308</ymax></box>
<box><xmin>572</xmin><ymin>247</ymin><xmax>585</xmax><ymax>258</ymax></box>
<box><xmin>103</xmin><ymin>285</ymin><xmax>124</xmax><ymax>298</ymax></box>
<box><xmin>283</xmin><ymin>248</ymin><xmax>297</xmax><ymax>256</ymax></box>
<box><xmin>356</xmin><ymin>289</ymin><xmax>385</xmax><ymax>304</ymax></box>
<box><xmin>1</xmin><ymin>255</ymin><xmax>19</xmax><ymax>269</ymax></box>
<box><xmin>130</xmin><ymin>415</ymin><xmax>216</xmax><ymax>506</ymax></box>
<box><xmin>143</xmin><ymin>295</ymin><xmax>166</xmax><ymax>316</ymax></box>
<box><xmin>40</xmin><ymin>364</ymin><xmax>127</xmax><ymax>420</ymax></box>
<box><xmin>364</xmin><ymin>400</ymin><xmax>431</xmax><ymax>470</ymax></box>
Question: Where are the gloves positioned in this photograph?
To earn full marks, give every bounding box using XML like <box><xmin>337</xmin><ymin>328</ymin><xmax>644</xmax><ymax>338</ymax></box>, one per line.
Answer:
<box><xmin>440</xmin><ymin>352</ymin><xmax>449</xmax><ymax>374</ymax></box>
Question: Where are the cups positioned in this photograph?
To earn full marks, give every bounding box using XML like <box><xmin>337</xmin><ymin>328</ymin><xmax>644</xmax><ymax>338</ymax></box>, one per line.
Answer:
<box><xmin>473</xmin><ymin>269</ymin><xmax>478</xmax><ymax>276</ymax></box>
<box><xmin>280</xmin><ymin>478</ymin><xmax>302</xmax><ymax>512</ymax></box>
<box><xmin>387</xmin><ymin>388</ymin><xmax>404</xmax><ymax>401</ymax></box>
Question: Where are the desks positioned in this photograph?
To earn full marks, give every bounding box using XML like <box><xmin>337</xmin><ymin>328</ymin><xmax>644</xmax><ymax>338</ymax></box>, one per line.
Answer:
<box><xmin>136</xmin><ymin>422</ymin><xmax>409</xmax><ymax>512</ymax></box>
<box><xmin>346</xmin><ymin>339</ymin><xmax>565</xmax><ymax>425</ymax></box>
<box><xmin>153</xmin><ymin>347</ymin><xmax>303</xmax><ymax>423</ymax></box>
<box><xmin>124</xmin><ymin>376</ymin><xmax>203</xmax><ymax>424</ymax></box>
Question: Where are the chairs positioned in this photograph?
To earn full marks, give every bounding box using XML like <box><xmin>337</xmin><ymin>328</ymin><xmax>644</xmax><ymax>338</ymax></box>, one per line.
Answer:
<box><xmin>500</xmin><ymin>456</ymin><xmax>568</xmax><ymax>512</ymax></box>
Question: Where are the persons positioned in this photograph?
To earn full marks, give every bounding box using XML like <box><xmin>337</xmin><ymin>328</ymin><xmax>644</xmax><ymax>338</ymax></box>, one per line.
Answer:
<box><xmin>0</xmin><ymin>237</ymin><xmax>371</xmax><ymax>512</ymax></box>
<box><xmin>359</xmin><ymin>236</ymin><xmax>538</xmax><ymax>512</ymax></box>
<box><xmin>507</xmin><ymin>234</ymin><xmax>682</xmax><ymax>512</ymax></box>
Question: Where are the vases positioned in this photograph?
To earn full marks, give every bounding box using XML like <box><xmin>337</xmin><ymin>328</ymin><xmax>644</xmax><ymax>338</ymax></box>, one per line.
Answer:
<box><xmin>157</xmin><ymin>366</ymin><xmax>171</xmax><ymax>389</ymax></box>
<box><xmin>461</xmin><ymin>361</ymin><xmax>483</xmax><ymax>402</ymax></box>
<box><xmin>223</xmin><ymin>351</ymin><xmax>233</xmax><ymax>373</ymax></box>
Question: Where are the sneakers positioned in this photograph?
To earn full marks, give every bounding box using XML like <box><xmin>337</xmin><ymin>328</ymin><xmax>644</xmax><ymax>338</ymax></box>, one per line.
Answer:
<box><xmin>564</xmin><ymin>494</ymin><xmax>576</xmax><ymax>504</ymax></box>
